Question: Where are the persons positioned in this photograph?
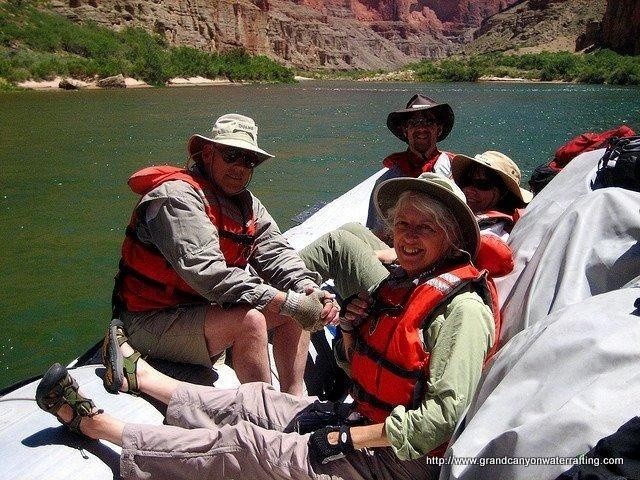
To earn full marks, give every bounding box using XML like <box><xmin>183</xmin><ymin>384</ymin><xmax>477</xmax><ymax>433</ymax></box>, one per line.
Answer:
<box><xmin>298</xmin><ymin>148</ymin><xmax>534</xmax><ymax>303</ymax></box>
<box><xmin>36</xmin><ymin>173</ymin><xmax>514</xmax><ymax>480</ymax></box>
<box><xmin>112</xmin><ymin>114</ymin><xmax>341</xmax><ymax>397</ymax></box>
<box><xmin>364</xmin><ymin>94</ymin><xmax>457</xmax><ymax>244</ymax></box>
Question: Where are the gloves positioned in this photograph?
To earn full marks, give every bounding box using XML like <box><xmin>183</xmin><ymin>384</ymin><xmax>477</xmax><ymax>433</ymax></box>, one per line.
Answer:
<box><xmin>306</xmin><ymin>424</ymin><xmax>354</xmax><ymax>465</ymax></box>
<box><xmin>280</xmin><ymin>279</ymin><xmax>375</xmax><ymax>334</ymax></box>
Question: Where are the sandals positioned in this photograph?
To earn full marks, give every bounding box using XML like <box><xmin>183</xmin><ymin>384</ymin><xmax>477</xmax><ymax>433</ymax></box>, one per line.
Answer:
<box><xmin>104</xmin><ymin>318</ymin><xmax>143</xmax><ymax>394</ymax></box>
<box><xmin>36</xmin><ymin>364</ymin><xmax>101</xmax><ymax>436</ymax></box>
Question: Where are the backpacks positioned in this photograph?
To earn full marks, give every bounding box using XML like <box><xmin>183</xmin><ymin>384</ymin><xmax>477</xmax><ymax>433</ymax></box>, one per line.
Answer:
<box><xmin>529</xmin><ymin>125</ymin><xmax>637</xmax><ymax>194</ymax></box>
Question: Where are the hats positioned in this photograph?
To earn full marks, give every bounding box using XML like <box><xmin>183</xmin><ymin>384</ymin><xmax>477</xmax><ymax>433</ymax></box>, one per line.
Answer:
<box><xmin>452</xmin><ymin>149</ymin><xmax>534</xmax><ymax>205</ymax></box>
<box><xmin>372</xmin><ymin>171</ymin><xmax>480</xmax><ymax>262</ymax></box>
<box><xmin>187</xmin><ymin>112</ymin><xmax>276</xmax><ymax>168</ymax></box>
<box><xmin>386</xmin><ymin>93</ymin><xmax>455</xmax><ymax>144</ymax></box>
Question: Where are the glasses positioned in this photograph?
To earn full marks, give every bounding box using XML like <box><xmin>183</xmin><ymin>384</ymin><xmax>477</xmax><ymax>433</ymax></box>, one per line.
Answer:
<box><xmin>458</xmin><ymin>171</ymin><xmax>499</xmax><ymax>192</ymax></box>
<box><xmin>208</xmin><ymin>145</ymin><xmax>262</xmax><ymax>170</ymax></box>
<box><xmin>402</xmin><ymin>117</ymin><xmax>439</xmax><ymax>128</ymax></box>
